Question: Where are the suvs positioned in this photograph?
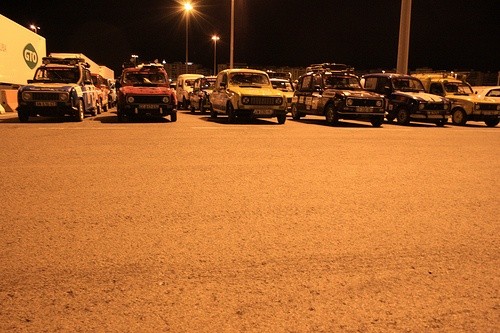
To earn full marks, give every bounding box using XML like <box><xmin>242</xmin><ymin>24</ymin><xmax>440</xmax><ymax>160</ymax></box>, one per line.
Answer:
<box><xmin>292</xmin><ymin>63</ymin><xmax>388</xmax><ymax>128</ymax></box>
<box><xmin>360</xmin><ymin>73</ymin><xmax>452</xmax><ymax>128</ymax></box>
<box><xmin>410</xmin><ymin>73</ymin><xmax>500</xmax><ymax>127</ymax></box>
<box><xmin>90</xmin><ymin>73</ymin><xmax>109</xmax><ymax>114</ymax></box>
<box><xmin>108</xmin><ymin>81</ymin><xmax>118</xmax><ymax>107</ymax></box>
<box><xmin>16</xmin><ymin>57</ymin><xmax>98</xmax><ymax>122</ymax></box>
<box><xmin>116</xmin><ymin>64</ymin><xmax>178</xmax><ymax>123</ymax></box>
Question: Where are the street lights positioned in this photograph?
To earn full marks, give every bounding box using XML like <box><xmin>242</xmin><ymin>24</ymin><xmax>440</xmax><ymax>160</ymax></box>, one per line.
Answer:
<box><xmin>212</xmin><ymin>33</ymin><xmax>220</xmax><ymax>76</ymax></box>
<box><xmin>30</xmin><ymin>25</ymin><xmax>40</xmax><ymax>34</ymax></box>
<box><xmin>183</xmin><ymin>0</ymin><xmax>192</xmax><ymax>72</ymax></box>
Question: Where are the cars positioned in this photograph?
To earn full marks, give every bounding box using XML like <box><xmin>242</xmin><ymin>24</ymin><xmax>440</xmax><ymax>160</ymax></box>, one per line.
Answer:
<box><xmin>269</xmin><ymin>78</ymin><xmax>296</xmax><ymax>115</ymax></box>
<box><xmin>190</xmin><ymin>76</ymin><xmax>217</xmax><ymax>114</ymax></box>
<box><xmin>210</xmin><ymin>68</ymin><xmax>288</xmax><ymax>125</ymax></box>
<box><xmin>478</xmin><ymin>87</ymin><xmax>500</xmax><ymax>98</ymax></box>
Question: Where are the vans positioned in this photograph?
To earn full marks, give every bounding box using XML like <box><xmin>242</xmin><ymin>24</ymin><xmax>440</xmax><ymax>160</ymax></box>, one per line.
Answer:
<box><xmin>176</xmin><ymin>73</ymin><xmax>203</xmax><ymax>110</ymax></box>
